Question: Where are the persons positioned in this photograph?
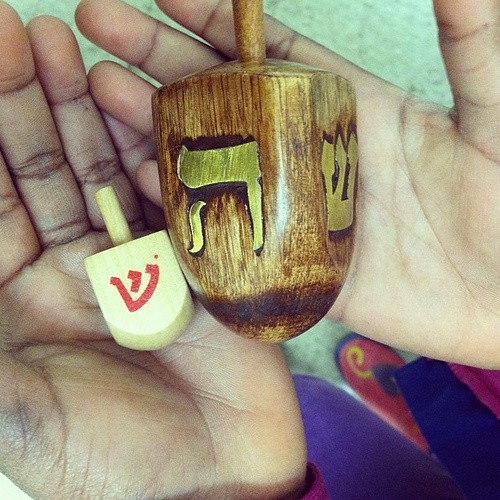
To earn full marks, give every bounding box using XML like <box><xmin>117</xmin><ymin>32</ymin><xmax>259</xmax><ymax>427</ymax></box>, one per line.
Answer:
<box><xmin>0</xmin><ymin>0</ymin><xmax>500</xmax><ymax>500</ymax></box>
